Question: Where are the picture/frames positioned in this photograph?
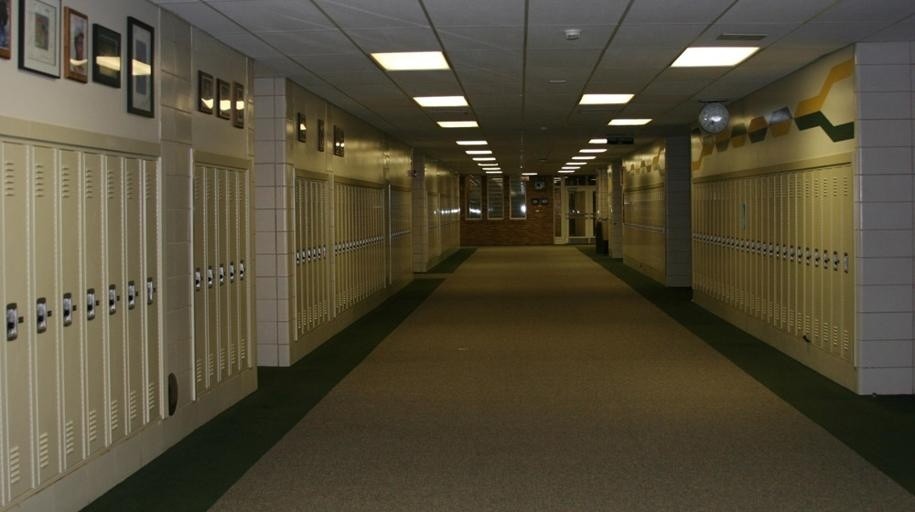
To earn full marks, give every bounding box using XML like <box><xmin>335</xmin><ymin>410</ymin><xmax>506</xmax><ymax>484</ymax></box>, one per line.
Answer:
<box><xmin>18</xmin><ymin>0</ymin><xmax>61</xmax><ymax>78</ymax></box>
<box><xmin>64</xmin><ymin>6</ymin><xmax>88</xmax><ymax>83</ymax></box>
<box><xmin>0</xmin><ymin>0</ymin><xmax>11</xmax><ymax>59</ymax></box>
<box><xmin>318</xmin><ymin>119</ymin><xmax>324</xmax><ymax>152</ymax></box>
<box><xmin>464</xmin><ymin>174</ymin><xmax>527</xmax><ymax>221</ymax></box>
<box><xmin>333</xmin><ymin>125</ymin><xmax>344</xmax><ymax>158</ymax></box>
<box><xmin>93</xmin><ymin>23</ymin><xmax>121</xmax><ymax>88</ymax></box>
<box><xmin>231</xmin><ymin>80</ymin><xmax>244</xmax><ymax>128</ymax></box>
<box><xmin>298</xmin><ymin>112</ymin><xmax>307</xmax><ymax>142</ymax></box>
<box><xmin>216</xmin><ymin>78</ymin><xmax>232</xmax><ymax>120</ymax></box>
<box><xmin>198</xmin><ymin>71</ymin><xmax>214</xmax><ymax>114</ymax></box>
<box><xmin>127</xmin><ymin>16</ymin><xmax>154</xmax><ymax>118</ymax></box>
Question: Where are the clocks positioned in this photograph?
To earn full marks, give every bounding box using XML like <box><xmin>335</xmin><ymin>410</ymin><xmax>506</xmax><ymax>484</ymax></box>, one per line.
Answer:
<box><xmin>697</xmin><ymin>100</ymin><xmax>730</xmax><ymax>135</ymax></box>
